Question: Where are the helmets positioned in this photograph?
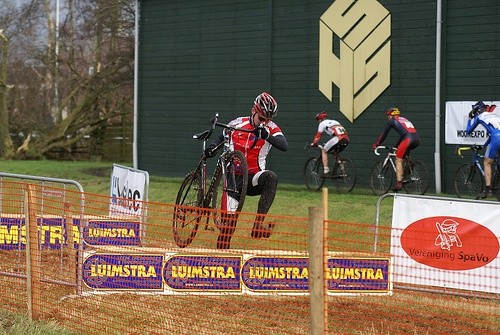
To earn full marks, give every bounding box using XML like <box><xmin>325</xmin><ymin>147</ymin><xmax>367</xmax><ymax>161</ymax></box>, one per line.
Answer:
<box><xmin>316</xmin><ymin>111</ymin><xmax>328</xmax><ymax>120</ymax></box>
<box><xmin>472</xmin><ymin>102</ymin><xmax>486</xmax><ymax>112</ymax></box>
<box><xmin>254</xmin><ymin>92</ymin><xmax>278</xmax><ymax>118</ymax></box>
<box><xmin>387</xmin><ymin>107</ymin><xmax>400</xmax><ymax>115</ymax></box>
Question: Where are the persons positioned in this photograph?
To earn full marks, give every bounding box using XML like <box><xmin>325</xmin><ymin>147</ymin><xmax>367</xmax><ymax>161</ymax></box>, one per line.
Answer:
<box><xmin>200</xmin><ymin>92</ymin><xmax>288</xmax><ymax>249</ymax></box>
<box><xmin>466</xmin><ymin>102</ymin><xmax>500</xmax><ymax>198</ymax></box>
<box><xmin>372</xmin><ymin>107</ymin><xmax>420</xmax><ymax>192</ymax></box>
<box><xmin>310</xmin><ymin>111</ymin><xmax>349</xmax><ymax>178</ymax></box>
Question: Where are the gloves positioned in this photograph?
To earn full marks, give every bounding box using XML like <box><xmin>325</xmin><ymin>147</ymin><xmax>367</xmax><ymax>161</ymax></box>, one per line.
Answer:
<box><xmin>469</xmin><ymin>111</ymin><xmax>474</xmax><ymax>118</ymax></box>
<box><xmin>205</xmin><ymin>143</ymin><xmax>217</xmax><ymax>157</ymax></box>
<box><xmin>254</xmin><ymin>126</ymin><xmax>268</xmax><ymax>139</ymax></box>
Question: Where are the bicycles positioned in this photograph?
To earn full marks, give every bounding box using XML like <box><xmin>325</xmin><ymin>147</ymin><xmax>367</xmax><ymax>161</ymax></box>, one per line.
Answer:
<box><xmin>453</xmin><ymin>144</ymin><xmax>500</xmax><ymax>201</ymax></box>
<box><xmin>368</xmin><ymin>141</ymin><xmax>430</xmax><ymax>197</ymax></box>
<box><xmin>303</xmin><ymin>139</ymin><xmax>358</xmax><ymax>195</ymax></box>
<box><xmin>172</xmin><ymin>112</ymin><xmax>264</xmax><ymax>249</ymax></box>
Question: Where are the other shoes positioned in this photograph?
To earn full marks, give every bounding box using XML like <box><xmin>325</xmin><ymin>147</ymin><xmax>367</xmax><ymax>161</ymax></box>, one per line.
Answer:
<box><xmin>478</xmin><ymin>190</ymin><xmax>492</xmax><ymax>198</ymax></box>
<box><xmin>250</xmin><ymin>227</ymin><xmax>271</xmax><ymax>238</ymax></box>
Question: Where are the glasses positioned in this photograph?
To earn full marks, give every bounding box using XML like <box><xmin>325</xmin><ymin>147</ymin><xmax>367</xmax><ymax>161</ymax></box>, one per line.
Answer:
<box><xmin>258</xmin><ymin>113</ymin><xmax>271</xmax><ymax>122</ymax></box>
